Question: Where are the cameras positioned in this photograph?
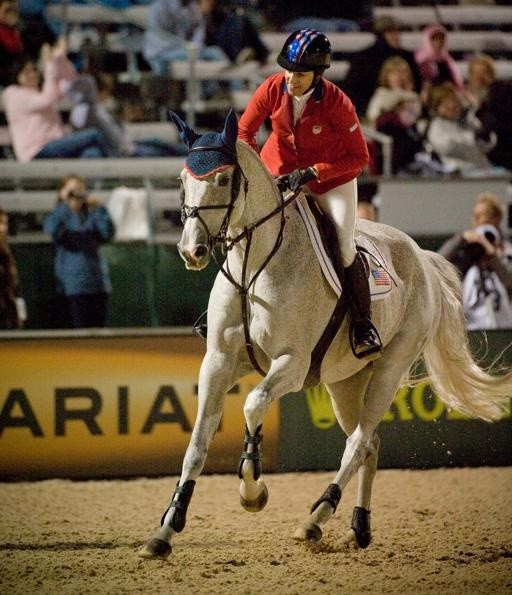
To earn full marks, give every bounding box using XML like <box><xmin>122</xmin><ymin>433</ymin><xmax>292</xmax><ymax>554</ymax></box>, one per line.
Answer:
<box><xmin>69</xmin><ymin>188</ymin><xmax>88</xmax><ymax>201</ymax></box>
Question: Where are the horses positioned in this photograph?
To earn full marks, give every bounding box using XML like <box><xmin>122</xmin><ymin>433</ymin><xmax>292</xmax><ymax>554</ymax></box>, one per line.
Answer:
<box><xmin>139</xmin><ymin>108</ymin><xmax>512</xmax><ymax>559</ymax></box>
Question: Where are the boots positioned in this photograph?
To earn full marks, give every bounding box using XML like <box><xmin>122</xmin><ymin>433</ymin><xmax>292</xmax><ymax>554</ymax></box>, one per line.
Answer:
<box><xmin>343</xmin><ymin>252</ymin><xmax>380</xmax><ymax>346</ymax></box>
<box><xmin>196</xmin><ymin>322</ymin><xmax>207</xmax><ymax>336</ymax></box>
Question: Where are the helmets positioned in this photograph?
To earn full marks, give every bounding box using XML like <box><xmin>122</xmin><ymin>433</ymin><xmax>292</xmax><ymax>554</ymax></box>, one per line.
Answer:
<box><xmin>277</xmin><ymin>29</ymin><xmax>331</xmax><ymax>72</ymax></box>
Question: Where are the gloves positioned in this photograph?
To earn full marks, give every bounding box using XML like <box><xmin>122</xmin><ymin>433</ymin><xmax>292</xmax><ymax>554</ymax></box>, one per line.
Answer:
<box><xmin>289</xmin><ymin>167</ymin><xmax>313</xmax><ymax>191</ymax></box>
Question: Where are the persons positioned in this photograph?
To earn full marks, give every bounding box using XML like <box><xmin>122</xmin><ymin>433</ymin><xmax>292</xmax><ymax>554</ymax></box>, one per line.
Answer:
<box><xmin>2</xmin><ymin>34</ymin><xmax>110</xmax><ymax>164</ymax></box>
<box><xmin>0</xmin><ymin>209</ymin><xmax>29</xmax><ymax>329</ymax></box>
<box><xmin>42</xmin><ymin>58</ymin><xmax>129</xmax><ymax>158</ymax></box>
<box><xmin>196</xmin><ymin>27</ymin><xmax>385</xmax><ymax>359</ymax></box>
<box><xmin>43</xmin><ymin>174</ymin><xmax>115</xmax><ymax>329</ymax></box>
<box><xmin>345</xmin><ymin>14</ymin><xmax>512</xmax><ymax>182</ymax></box>
<box><xmin>435</xmin><ymin>193</ymin><xmax>512</xmax><ymax>329</ymax></box>
<box><xmin>137</xmin><ymin>0</ymin><xmax>272</xmax><ymax>83</ymax></box>
